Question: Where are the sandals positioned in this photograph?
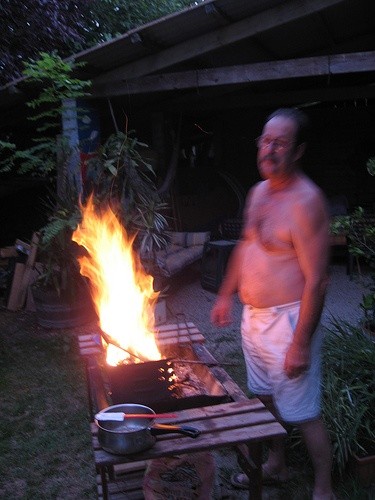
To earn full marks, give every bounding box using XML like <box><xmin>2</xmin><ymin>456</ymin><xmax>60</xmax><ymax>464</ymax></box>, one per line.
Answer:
<box><xmin>230</xmin><ymin>466</ymin><xmax>288</xmax><ymax>488</ymax></box>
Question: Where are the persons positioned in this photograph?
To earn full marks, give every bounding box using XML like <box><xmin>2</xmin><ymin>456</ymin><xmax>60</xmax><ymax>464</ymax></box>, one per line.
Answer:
<box><xmin>210</xmin><ymin>111</ymin><xmax>335</xmax><ymax>500</ymax></box>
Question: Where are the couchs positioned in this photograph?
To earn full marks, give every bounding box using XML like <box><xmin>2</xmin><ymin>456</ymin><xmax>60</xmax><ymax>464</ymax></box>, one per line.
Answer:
<box><xmin>136</xmin><ymin>228</ymin><xmax>210</xmax><ymax>292</ymax></box>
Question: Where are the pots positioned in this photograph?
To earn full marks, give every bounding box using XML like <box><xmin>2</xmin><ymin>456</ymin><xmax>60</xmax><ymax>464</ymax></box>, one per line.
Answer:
<box><xmin>95</xmin><ymin>404</ymin><xmax>201</xmax><ymax>455</ymax></box>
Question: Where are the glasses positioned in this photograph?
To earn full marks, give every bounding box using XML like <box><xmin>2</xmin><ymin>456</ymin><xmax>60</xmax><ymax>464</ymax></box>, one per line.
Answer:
<box><xmin>256</xmin><ymin>135</ymin><xmax>299</xmax><ymax>153</ymax></box>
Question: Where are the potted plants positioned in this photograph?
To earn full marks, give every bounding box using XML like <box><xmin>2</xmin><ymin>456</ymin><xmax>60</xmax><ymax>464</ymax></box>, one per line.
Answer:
<box><xmin>318</xmin><ymin>205</ymin><xmax>375</xmax><ymax>487</ymax></box>
<box><xmin>0</xmin><ymin>50</ymin><xmax>169</xmax><ymax>351</ymax></box>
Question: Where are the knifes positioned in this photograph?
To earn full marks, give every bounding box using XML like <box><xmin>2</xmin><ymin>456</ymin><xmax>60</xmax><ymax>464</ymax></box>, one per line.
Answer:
<box><xmin>95</xmin><ymin>412</ymin><xmax>178</xmax><ymax>421</ymax></box>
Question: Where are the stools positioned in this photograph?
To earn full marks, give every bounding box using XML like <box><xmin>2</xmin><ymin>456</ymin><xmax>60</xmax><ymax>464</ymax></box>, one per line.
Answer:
<box><xmin>200</xmin><ymin>239</ymin><xmax>243</xmax><ymax>296</ymax></box>
